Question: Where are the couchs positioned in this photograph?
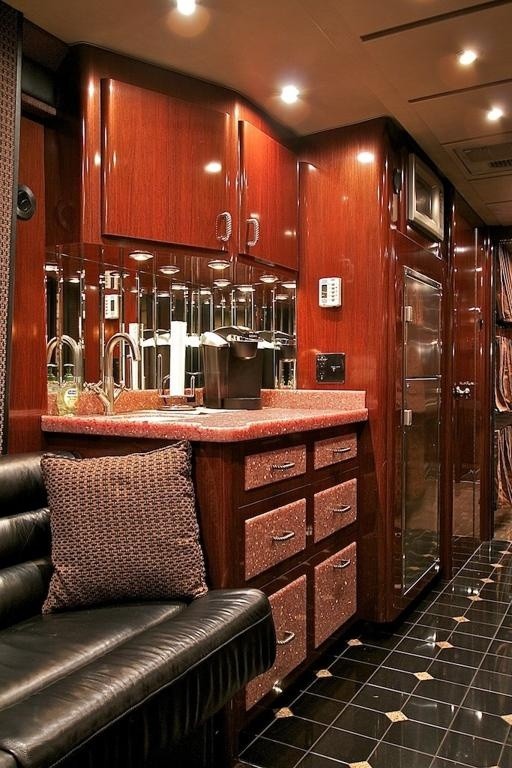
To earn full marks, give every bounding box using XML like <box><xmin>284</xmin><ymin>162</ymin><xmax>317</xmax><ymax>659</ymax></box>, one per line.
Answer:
<box><xmin>1</xmin><ymin>451</ymin><xmax>278</xmax><ymax>768</ymax></box>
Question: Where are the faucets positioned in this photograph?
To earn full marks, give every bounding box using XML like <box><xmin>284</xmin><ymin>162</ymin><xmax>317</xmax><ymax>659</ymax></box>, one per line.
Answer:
<box><xmin>47</xmin><ymin>331</ymin><xmax>84</xmax><ymax>386</ymax></box>
<box><xmin>91</xmin><ymin>331</ymin><xmax>142</xmax><ymax>415</ymax></box>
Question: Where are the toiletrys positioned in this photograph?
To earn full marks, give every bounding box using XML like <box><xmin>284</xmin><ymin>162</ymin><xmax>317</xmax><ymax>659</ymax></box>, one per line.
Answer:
<box><xmin>55</xmin><ymin>360</ymin><xmax>83</xmax><ymax>417</ymax></box>
